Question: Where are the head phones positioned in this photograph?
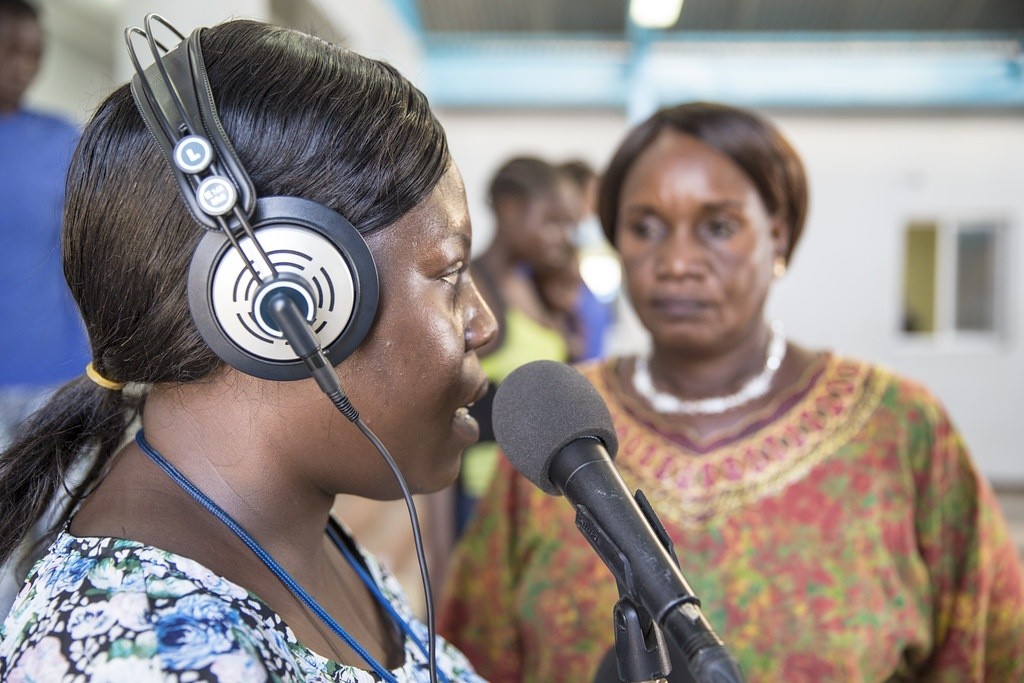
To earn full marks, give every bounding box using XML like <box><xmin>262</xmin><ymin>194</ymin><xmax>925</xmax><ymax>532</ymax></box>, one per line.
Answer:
<box><xmin>122</xmin><ymin>13</ymin><xmax>380</xmax><ymax>381</ymax></box>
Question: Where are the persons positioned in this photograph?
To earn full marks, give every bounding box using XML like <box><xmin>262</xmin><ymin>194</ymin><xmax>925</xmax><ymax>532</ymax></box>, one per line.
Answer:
<box><xmin>438</xmin><ymin>150</ymin><xmax>614</xmax><ymax>550</ymax></box>
<box><xmin>0</xmin><ymin>0</ymin><xmax>96</xmax><ymax>631</ymax></box>
<box><xmin>0</xmin><ymin>21</ymin><xmax>501</xmax><ymax>683</ymax></box>
<box><xmin>434</xmin><ymin>101</ymin><xmax>1024</xmax><ymax>683</ymax></box>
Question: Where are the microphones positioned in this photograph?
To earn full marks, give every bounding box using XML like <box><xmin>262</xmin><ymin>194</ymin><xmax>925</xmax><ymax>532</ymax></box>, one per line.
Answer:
<box><xmin>493</xmin><ymin>360</ymin><xmax>747</xmax><ymax>683</ymax></box>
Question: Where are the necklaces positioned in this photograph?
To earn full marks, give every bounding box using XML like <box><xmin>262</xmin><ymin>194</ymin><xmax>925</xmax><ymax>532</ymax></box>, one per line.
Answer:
<box><xmin>630</xmin><ymin>331</ymin><xmax>787</xmax><ymax>416</ymax></box>
<box><xmin>135</xmin><ymin>428</ymin><xmax>448</xmax><ymax>683</ymax></box>
